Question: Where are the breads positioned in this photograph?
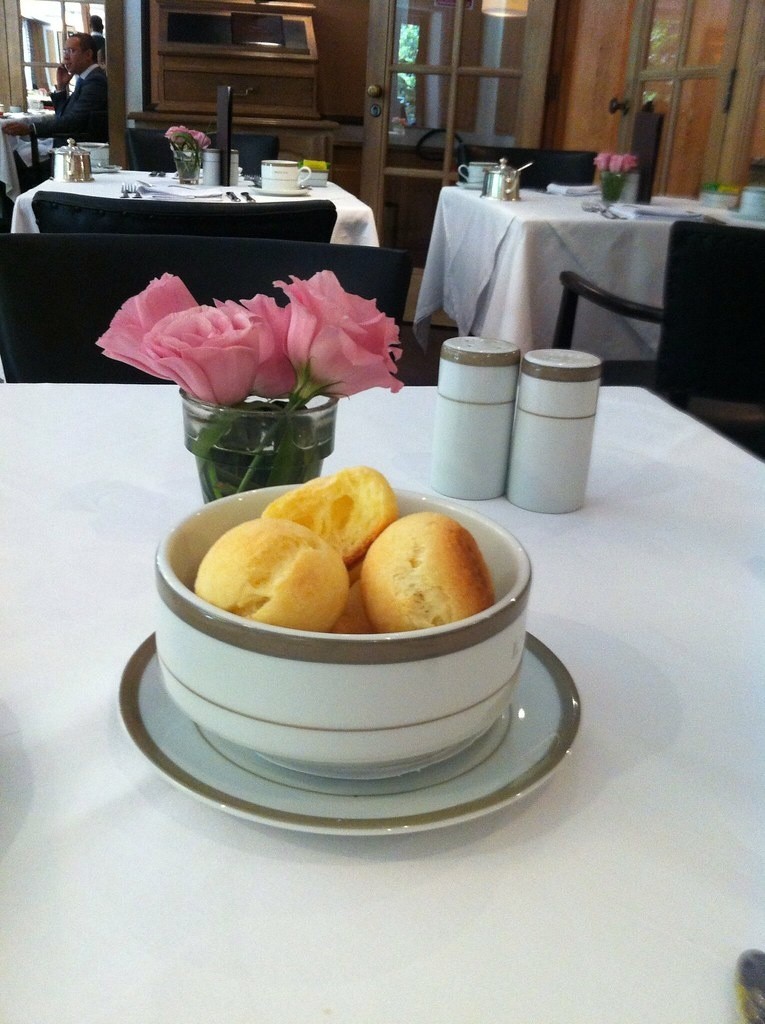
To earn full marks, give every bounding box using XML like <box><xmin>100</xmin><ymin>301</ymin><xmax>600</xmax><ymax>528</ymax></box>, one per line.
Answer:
<box><xmin>195</xmin><ymin>467</ymin><xmax>494</xmax><ymax>633</ymax></box>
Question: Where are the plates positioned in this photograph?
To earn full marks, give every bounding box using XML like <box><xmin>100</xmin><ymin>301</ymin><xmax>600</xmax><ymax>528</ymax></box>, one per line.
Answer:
<box><xmin>118</xmin><ymin>628</ymin><xmax>580</xmax><ymax>834</ymax></box>
<box><xmin>456</xmin><ymin>181</ymin><xmax>484</xmax><ymax>189</ymax></box>
<box><xmin>255</xmin><ymin>189</ymin><xmax>308</xmax><ymax>196</ymax></box>
<box><xmin>91</xmin><ymin>165</ymin><xmax>122</xmax><ymax>173</ymax></box>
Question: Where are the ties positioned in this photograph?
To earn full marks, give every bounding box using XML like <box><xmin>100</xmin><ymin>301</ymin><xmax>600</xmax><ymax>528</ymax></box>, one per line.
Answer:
<box><xmin>57</xmin><ymin>77</ymin><xmax>83</xmax><ymax>120</ymax></box>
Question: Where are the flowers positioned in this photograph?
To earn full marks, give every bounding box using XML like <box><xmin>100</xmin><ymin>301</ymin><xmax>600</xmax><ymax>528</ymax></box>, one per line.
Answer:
<box><xmin>164</xmin><ymin>125</ymin><xmax>212</xmax><ymax>150</ymax></box>
<box><xmin>592</xmin><ymin>150</ymin><xmax>638</xmax><ymax>173</ymax></box>
<box><xmin>94</xmin><ymin>268</ymin><xmax>407</xmax><ymax>494</ymax></box>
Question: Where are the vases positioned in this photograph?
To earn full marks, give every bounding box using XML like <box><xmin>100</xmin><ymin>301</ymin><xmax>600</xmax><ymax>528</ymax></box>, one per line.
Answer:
<box><xmin>172</xmin><ymin>150</ymin><xmax>202</xmax><ymax>184</ymax></box>
<box><xmin>176</xmin><ymin>383</ymin><xmax>339</xmax><ymax>504</ymax></box>
<box><xmin>600</xmin><ymin>172</ymin><xmax>626</xmax><ymax>202</ymax></box>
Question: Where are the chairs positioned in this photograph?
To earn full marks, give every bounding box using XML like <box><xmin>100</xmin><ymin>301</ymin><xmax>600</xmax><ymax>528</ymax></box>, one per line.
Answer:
<box><xmin>550</xmin><ymin>219</ymin><xmax>765</xmax><ymax>460</ymax></box>
<box><xmin>125</xmin><ymin>127</ymin><xmax>279</xmax><ymax>176</ymax></box>
<box><xmin>32</xmin><ymin>189</ymin><xmax>338</xmax><ymax>244</ymax></box>
<box><xmin>0</xmin><ymin>230</ymin><xmax>413</xmax><ymax>386</ymax></box>
<box><xmin>457</xmin><ymin>142</ymin><xmax>598</xmax><ymax>188</ymax></box>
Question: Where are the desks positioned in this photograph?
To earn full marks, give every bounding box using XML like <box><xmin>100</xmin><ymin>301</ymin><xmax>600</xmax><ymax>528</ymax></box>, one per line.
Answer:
<box><xmin>412</xmin><ymin>184</ymin><xmax>765</xmax><ymax>367</ymax></box>
<box><xmin>0</xmin><ymin>109</ymin><xmax>56</xmax><ymax>218</ymax></box>
<box><xmin>10</xmin><ymin>169</ymin><xmax>379</xmax><ymax>250</ymax></box>
<box><xmin>0</xmin><ymin>385</ymin><xmax>765</xmax><ymax>1024</ymax></box>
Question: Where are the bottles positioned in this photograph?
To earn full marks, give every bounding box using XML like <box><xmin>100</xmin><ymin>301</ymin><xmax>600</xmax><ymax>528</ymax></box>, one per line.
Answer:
<box><xmin>203</xmin><ymin>149</ymin><xmax>222</xmax><ymax>186</ymax></box>
<box><xmin>52</xmin><ymin>138</ymin><xmax>90</xmax><ymax>183</ymax></box>
<box><xmin>622</xmin><ymin>170</ymin><xmax>641</xmax><ymax>204</ymax></box>
<box><xmin>480</xmin><ymin>159</ymin><xmax>519</xmax><ymax>200</ymax></box>
<box><xmin>230</xmin><ymin>149</ymin><xmax>240</xmax><ymax>185</ymax></box>
<box><xmin>738</xmin><ymin>186</ymin><xmax>765</xmax><ymax>219</ymax></box>
<box><xmin>430</xmin><ymin>335</ymin><xmax>604</xmax><ymax>510</ymax></box>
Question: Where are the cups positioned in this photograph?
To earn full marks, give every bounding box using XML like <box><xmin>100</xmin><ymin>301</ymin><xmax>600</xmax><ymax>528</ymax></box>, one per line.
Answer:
<box><xmin>456</xmin><ymin>162</ymin><xmax>497</xmax><ymax>182</ymax></box>
<box><xmin>262</xmin><ymin>160</ymin><xmax>311</xmax><ymax>190</ymax></box>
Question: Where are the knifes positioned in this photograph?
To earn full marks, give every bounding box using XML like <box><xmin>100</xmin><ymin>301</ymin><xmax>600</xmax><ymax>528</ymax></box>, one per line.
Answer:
<box><xmin>687</xmin><ymin>208</ymin><xmax>728</xmax><ymax>225</ymax></box>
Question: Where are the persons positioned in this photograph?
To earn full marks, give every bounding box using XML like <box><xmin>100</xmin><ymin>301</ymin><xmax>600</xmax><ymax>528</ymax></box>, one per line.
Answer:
<box><xmin>2</xmin><ymin>33</ymin><xmax>109</xmax><ymax>148</ymax></box>
<box><xmin>88</xmin><ymin>15</ymin><xmax>106</xmax><ymax>65</ymax></box>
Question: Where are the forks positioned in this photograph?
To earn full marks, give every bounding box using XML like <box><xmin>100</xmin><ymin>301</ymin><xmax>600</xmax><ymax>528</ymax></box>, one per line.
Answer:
<box><xmin>122</xmin><ymin>183</ymin><xmax>142</xmax><ymax>198</ymax></box>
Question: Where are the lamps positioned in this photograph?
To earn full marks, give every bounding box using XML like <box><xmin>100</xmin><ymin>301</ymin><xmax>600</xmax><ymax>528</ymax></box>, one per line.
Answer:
<box><xmin>481</xmin><ymin>0</ymin><xmax>528</xmax><ymax>18</ymax></box>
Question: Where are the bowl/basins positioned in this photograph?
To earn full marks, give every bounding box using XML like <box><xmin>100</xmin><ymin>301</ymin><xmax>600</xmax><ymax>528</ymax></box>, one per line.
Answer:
<box><xmin>701</xmin><ymin>191</ymin><xmax>739</xmax><ymax>211</ymax></box>
<box><xmin>300</xmin><ymin>171</ymin><xmax>330</xmax><ymax>188</ymax></box>
<box><xmin>154</xmin><ymin>484</ymin><xmax>530</xmax><ymax>779</ymax></box>
<box><xmin>77</xmin><ymin>141</ymin><xmax>110</xmax><ymax>166</ymax></box>
<box><xmin>10</xmin><ymin>107</ymin><xmax>22</xmax><ymax>112</ymax></box>
